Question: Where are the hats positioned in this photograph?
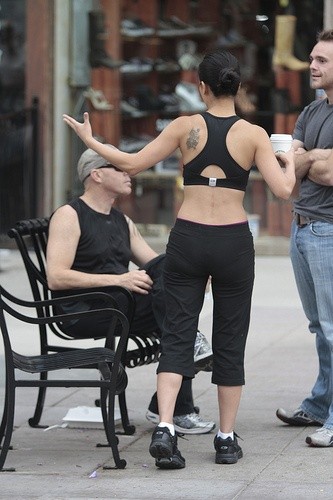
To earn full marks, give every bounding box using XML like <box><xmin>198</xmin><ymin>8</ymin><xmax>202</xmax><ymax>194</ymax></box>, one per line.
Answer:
<box><xmin>76</xmin><ymin>144</ymin><xmax>120</xmax><ymax>182</ymax></box>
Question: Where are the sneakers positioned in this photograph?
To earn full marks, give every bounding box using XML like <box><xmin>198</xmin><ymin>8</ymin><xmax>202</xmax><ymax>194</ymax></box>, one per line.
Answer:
<box><xmin>214</xmin><ymin>430</ymin><xmax>244</xmax><ymax>464</ymax></box>
<box><xmin>306</xmin><ymin>426</ymin><xmax>333</xmax><ymax>447</ymax></box>
<box><xmin>276</xmin><ymin>406</ymin><xmax>322</xmax><ymax>426</ymax></box>
<box><xmin>149</xmin><ymin>425</ymin><xmax>185</xmax><ymax>469</ymax></box>
<box><xmin>146</xmin><ymin>409</ymin><xmax>216</xmax><ymax>434</ymax></box>
<box><xmin>193</xmin><ymin>332</ymin><xmax>215</xmax><ymax>371</ymax></box>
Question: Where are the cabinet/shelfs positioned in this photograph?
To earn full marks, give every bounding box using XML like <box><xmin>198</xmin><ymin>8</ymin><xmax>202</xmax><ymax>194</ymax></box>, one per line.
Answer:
<box><xmin>84</xmin><ymin>0</ymin><xmax>262</xmax><ymax>223</ymax></box>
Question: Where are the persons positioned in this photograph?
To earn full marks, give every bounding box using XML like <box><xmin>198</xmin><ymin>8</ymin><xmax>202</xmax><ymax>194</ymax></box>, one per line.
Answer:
<box><xmin>276</xmin><ymin>29</ymin><xmax>333</xmax><ymax>448</ymax></box>
<box><xmin>46</xmin><ymin>143</ymin><xmax>216</xmax><ymax>435</ymax></box>
<box><xmin>63</xmin><ymin>50</ymin><xmax>296</xmax><ymax>469</ymax></box>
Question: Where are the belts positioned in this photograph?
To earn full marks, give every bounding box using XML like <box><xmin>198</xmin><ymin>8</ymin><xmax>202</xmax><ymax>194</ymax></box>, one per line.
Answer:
<box><xmin>293</xmin><ymin>211</ymin><xmax>319</xmax><ymax>228</ymax></box>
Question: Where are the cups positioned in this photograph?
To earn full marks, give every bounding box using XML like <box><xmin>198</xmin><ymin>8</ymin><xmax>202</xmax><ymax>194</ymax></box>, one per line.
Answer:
<box><xmin>269</xmin><ymin>133</ymin><xmax>293</xmax><ymax>167</ymax></box>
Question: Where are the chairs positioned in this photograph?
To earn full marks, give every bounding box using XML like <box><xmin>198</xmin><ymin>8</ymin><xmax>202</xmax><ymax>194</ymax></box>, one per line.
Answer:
<box><xmin>0</xmin><ymin>216</ymin><xmax>164</xmax><ymax>470</ymax></box>
